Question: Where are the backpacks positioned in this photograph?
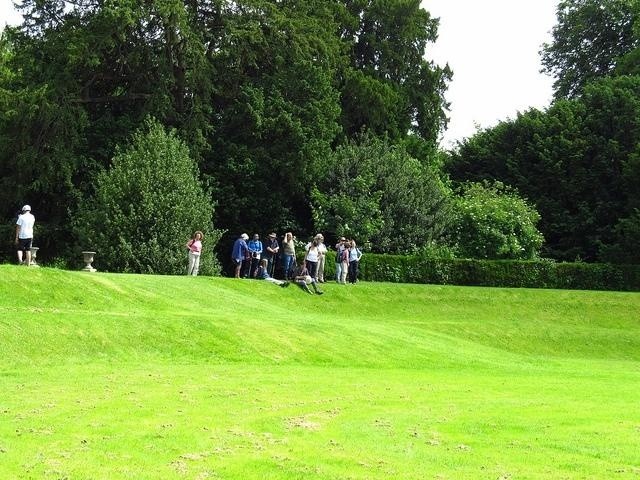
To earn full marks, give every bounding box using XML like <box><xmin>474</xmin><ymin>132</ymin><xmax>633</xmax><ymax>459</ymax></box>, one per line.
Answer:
<box><xmin>335</xmin><ymin>250</ymin><xmax>347</xmax><ymax>263</ymax></box>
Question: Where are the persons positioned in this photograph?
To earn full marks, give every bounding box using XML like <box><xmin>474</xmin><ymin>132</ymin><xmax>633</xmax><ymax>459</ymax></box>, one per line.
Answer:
<box><xmin>253</xmin><ymin>258</ymin><xmax>290</xmax><ymax>287</ymax></box>
<box><xmin>186</xmin><ymin>231</ymin><xmax>203</xmax><ymax>276</ymax></box>
<box><xmin>263</xmin><ymin>232</ymin><xmax>280</xmax><ymax>278</ymax></box>
<box><xmin>304</xmin><ymin>233</ymin><xmax>362</xmax><ymax>285</ymax></box>
<box><xmin>243</xmin><ymin>233</ymin><xmax>263</xmax><ymax>278</ymax></box>
<box><xmin>232</xmin><ymin>233</ymin><xmax>250</xmax><ymax>278</ymax></box>
<box><xmin>14</xmin><ymin>205</ymin><xmax>36</xmax><ymax>266</ymax></box>
<box><xmin>293</xmin><ymin>261</ymin><xmax>324</xmax><ymax>294</ymax></box>
<box><xmin>282</xmin><ymin>232</ymin><xmax>296</xmax><ymax>280</ymax></box>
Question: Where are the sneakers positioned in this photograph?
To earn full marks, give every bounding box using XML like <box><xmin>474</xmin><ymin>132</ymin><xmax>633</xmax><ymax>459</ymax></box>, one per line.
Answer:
<box><xmin>308</xmin><ymin>289</ymin><xmax>323</xmax><ymax>295</ymax></box>
<box><xmin>281</xmin><ymin>281</ymin><xmax>290</xmax><ymax>287</ymax></box>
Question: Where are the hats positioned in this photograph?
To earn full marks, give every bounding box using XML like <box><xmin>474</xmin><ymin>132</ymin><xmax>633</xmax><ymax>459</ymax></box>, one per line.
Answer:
<box><xmin>269</xmin><ymin>233</ymin><xmax>276</xmax><ymax>238</ymax></box>
<box><xmin>22</xmin><ymin>205</ymin><xmax>31</xmax><ymax>211</ymax></box>
<box><xmin>193</xmin><ymin>231</ymin><xmax>204</xmax><ymax>240</ymax></box>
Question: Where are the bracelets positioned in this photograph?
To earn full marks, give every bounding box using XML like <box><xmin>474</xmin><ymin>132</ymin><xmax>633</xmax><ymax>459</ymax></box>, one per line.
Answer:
<box><xmin>15</xmin><ymin>237</ymin><xmax>18</xmax><ymax>238</ymax></box>
